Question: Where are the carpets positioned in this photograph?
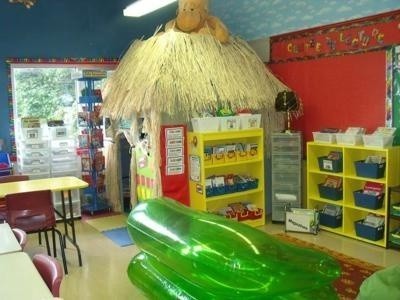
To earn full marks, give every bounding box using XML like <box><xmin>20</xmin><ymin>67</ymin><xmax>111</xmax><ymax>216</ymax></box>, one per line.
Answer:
<box><xmin>81</xmin><ymin>212</ymin><xmax>135</xmax><ymax>247</ymax></box>
<box><xmin>272</xmin><ymin>233</ymin><xmax>386</xmax><ymax>300</ymax></box>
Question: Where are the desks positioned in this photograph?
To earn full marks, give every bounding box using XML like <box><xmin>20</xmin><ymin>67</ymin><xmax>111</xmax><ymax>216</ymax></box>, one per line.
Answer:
<box><xmin>0</xmin><ymin>176</ymin><xmax>92</xmax><ymax>266</ymax></box>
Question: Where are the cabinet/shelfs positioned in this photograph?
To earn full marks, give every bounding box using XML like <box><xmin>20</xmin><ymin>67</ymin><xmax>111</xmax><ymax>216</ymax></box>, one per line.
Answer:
<box><xmin>15</xmin><ymin>125</ymin><xmax>82</xmax><ymax>222</ymax></box>
<box><xmin>74</xmin><ymin>76</ymin><xmax>112</xmax><ymax>216</ymax></box>
<box><xmin>187</xmin><ymin>128</ymin><xmax>399</xmax><ymax>248</ymax></box>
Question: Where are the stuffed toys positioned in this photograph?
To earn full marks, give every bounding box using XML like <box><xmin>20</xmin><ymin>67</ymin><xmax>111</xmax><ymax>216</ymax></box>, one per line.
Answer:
<box><xmin>155</xmin><ymin>1</ymin><xmax>230</xmax><ymax>44</ymax></box>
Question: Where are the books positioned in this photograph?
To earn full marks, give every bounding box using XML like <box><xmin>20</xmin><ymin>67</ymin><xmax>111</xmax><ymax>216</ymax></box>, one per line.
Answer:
<box><xmin>19</xmin><ymin>116</ymin><xmax>67</xmax><ymax>137</ymax></box>
<box><xmin>314</xmin><ymin>125</ymin><xmax>397</xmax><ymax>229</ymax></box>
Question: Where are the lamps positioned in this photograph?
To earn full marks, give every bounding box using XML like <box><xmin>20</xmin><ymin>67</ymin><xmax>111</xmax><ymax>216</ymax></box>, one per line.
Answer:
<box><xmin>275</xmin><ymin>89</ymin><xmax>301</xmax><ymax>133</ymax></box>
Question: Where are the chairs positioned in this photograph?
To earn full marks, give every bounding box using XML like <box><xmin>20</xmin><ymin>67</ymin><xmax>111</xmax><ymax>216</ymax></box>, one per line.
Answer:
<box><xmin>0</xmin><ymin>173</ymin><xmax>71</xmax><ymax>300</ymax></box>
<box><xmin>126</xmin><ymin>196</ymin><xmax>343</xmax><ymax>300</ymax></box>
